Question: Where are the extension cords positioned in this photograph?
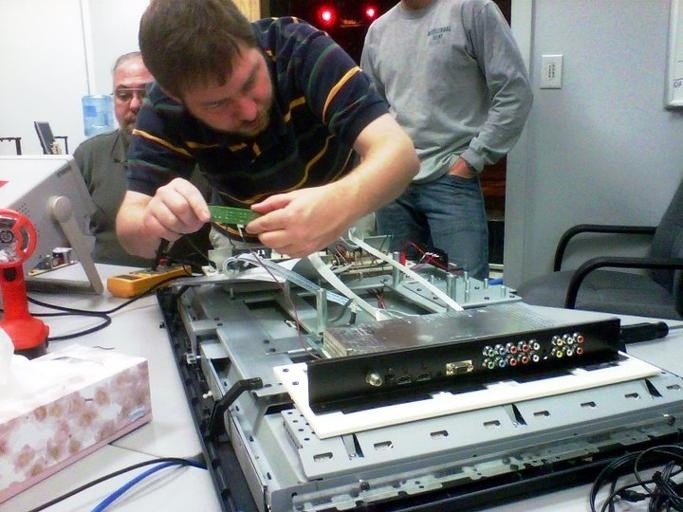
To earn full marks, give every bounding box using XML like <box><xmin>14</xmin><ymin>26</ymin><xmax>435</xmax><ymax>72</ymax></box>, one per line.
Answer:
<box><xmin>107</xmin><ymin>262</ymin><xmax>192</xmax><ymax>297</ymax></box>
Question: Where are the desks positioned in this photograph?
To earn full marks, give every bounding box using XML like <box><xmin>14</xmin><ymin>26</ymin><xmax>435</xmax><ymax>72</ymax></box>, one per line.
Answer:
<box><xmin>0</xmin><ymin>257</ymin><xmax>683</xmax><ymax>511</ymax></box>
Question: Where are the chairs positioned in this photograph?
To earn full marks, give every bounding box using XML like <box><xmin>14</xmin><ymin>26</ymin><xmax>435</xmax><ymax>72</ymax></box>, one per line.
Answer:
<box><xmin>513</xmin><ymin>176</ymin><xmax>682</xmax><ymax>318</ymax></box>
<box><xmin>34</xmin><ymin>119</ymin><xmax>69</xmax><ymax>157</ymax></box>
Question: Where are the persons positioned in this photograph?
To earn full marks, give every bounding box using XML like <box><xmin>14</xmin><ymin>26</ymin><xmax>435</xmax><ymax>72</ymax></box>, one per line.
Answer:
<box><xmin>358</xmin><ymin>0</ymin><xmax>535</xmax><ymax>285</ymax></box>
<box><xmin>72</xmin><ymin>51</ymin><xmax>224</xmax><ymax>274</ymax></box>
<box><xmin>114</xmin><ymin>0</ymin><xmax>421</xmax><ymax>268</ymax></box>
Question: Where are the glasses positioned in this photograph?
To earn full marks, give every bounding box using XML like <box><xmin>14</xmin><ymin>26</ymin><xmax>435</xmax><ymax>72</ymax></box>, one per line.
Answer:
<box><xmin>108</xmin><ymin>86</ymin><xmax>149</xmax><ymax>104</ymax></box>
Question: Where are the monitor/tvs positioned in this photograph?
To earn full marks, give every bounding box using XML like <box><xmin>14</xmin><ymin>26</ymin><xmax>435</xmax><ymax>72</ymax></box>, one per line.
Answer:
<box><xmin>34</xmin><ymin>121</ymin><xmax>55</xmax><ymax>154</ymax></box>
<box><xmin>150</xmin><ymin>232</ymin><xmax>682</xmax><ymax>512</ymax></box>
